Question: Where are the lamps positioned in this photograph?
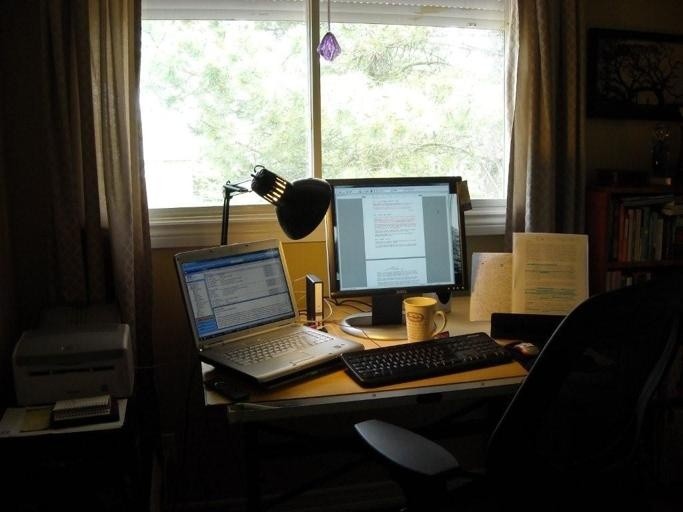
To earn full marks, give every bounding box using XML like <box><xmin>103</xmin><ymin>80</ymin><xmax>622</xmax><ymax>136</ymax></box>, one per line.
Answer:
<box><xmin>219</xmin><ymin>162</ymin><xmax>331</xmax><ymax>245</ymax></box>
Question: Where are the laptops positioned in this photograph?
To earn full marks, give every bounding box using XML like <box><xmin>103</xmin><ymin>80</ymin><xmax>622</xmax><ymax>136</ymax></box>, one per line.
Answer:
<box><xmin>173</xmin><ymin>237</ymin><xmax>365</xmax><ymax>389</ymax></box>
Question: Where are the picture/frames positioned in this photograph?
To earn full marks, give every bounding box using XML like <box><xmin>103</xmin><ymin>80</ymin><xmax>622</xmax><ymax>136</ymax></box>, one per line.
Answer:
<box><xmin>585</xmin><ymin>27</ymin><xmax>682</xmax><ymax>122</ymax></box>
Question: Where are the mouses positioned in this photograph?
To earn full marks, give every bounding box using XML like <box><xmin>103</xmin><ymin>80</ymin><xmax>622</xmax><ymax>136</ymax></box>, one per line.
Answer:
<box><xmin>514</xmin><ymin>342</ymin><xmax>539</xmax><ymax>357</ymax></box>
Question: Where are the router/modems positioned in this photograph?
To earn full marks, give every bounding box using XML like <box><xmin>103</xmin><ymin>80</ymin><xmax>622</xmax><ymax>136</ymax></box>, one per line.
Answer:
<box><xmin>306</xmin><ymin>273</ymin><xmax>324</xmax><ymax>332</ymax></box>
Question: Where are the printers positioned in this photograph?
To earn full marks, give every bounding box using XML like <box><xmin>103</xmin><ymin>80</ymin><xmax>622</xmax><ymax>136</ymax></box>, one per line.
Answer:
<box><xmin>12</xmin><ymin>323</ymin><xmax>135</xmax><ymax>408</ymax></box>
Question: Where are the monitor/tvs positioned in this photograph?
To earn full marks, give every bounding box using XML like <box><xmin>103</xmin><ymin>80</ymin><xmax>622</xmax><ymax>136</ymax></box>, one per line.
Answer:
<box><xmin>327</xmin><ymin>176</ymin><xmax>471</xmax><ymax>339</ymax></box>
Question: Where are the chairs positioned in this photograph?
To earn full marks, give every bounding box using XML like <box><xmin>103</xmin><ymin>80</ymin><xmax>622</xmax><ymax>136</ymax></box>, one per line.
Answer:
<box><xmin>354</xmin><ymin>282</ymin><xmax>682</xmax><ymax>511</ymax></box>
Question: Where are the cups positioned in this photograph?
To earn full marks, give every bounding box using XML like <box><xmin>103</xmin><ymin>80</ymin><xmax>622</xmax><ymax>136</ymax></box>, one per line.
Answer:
<box><xmin>404</xmin><ymin>296</ymin><xmax>447</xmax><ymax>342</ymax></box>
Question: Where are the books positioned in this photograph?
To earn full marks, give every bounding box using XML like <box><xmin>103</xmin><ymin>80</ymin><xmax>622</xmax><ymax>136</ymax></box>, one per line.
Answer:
<box><xmin>586</xmin><ymin>184</ymin><xmax>683</xmax><ymax>296</ymax></box>
<box><xmin>48</xmin><ymin>400</ymin><xmax>119</xmax><ymax>427</ymax></box>
<box><xmin>511</xmin><ymin>231</ymin><xmax>590</xmax><ymax>315</ymax></box>
<box><xmin>469</xmin><ymin>252</ymin><xmax>514</xmax><ymax>322</ymax></box>
<box><xmin>53</xmin><ymin>392</ymin><xmax>111</xmax><ymax>421</ymax></box>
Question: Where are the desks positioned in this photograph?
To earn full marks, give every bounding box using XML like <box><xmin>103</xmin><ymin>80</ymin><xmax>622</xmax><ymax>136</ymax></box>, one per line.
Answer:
<box><xmin>199</xmin><ymin>292</ymin><xmax>552</xmax><ymax>510</ymax></box>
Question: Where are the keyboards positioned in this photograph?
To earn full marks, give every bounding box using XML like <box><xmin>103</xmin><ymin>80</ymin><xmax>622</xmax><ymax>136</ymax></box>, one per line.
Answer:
<box><xmin>340</xmin><ymin>332</ymin><xmax>514</xmax><ymax>387</ymax></box>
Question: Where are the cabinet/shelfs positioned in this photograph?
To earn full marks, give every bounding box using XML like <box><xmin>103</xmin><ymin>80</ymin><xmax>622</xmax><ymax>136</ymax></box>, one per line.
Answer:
<box><xmin>587</xmin><ymin>186</ymin><xmax>681</xmax><ymax>292</ymax></box>
